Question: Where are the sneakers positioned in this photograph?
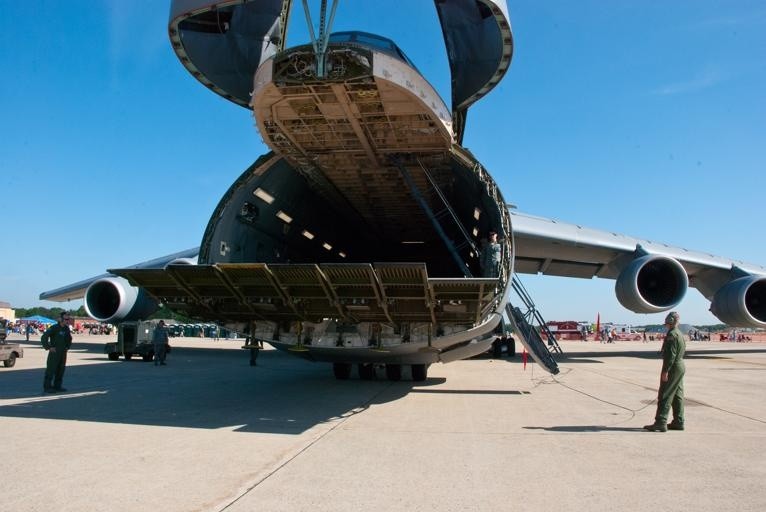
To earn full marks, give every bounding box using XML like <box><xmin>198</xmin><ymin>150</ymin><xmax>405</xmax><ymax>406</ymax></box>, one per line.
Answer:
<box><xmin>643</xmin><ymin>423</ymin><xmax>667</xmax><ymax>432</ymax></box>
<box><xmin>44</xmin><ymin>386</ymin><xmax>67</xmax><ymax>393</ymax></box>
<box><xmin>667</xmin><ymin>423</ymin><xmax>684</xmax><ymax>430</ymax></box>
<box><xmin>154</xmin><ymin>362</ymin><xmax>168</xmax><ymax>367</ymax></box>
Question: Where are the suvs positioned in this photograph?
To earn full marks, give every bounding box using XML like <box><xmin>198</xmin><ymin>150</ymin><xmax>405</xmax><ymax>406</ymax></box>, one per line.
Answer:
<box><xmin>0</xmin><ymin>337</ymin><xmax>22</xmax><ymax>367</ymax></box>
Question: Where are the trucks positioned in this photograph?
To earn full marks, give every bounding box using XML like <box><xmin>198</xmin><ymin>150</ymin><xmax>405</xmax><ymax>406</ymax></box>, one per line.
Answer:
<box><xmin>0</xmin><ymin>317</ymin><xmax>11</xmax><ymax>339</ymax></box>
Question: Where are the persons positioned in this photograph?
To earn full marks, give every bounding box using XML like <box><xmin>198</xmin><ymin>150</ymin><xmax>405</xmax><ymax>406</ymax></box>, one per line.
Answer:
<box><xmin>720</xmin><ymin>329</ymin><xmax>751</xmax><ymax>343</ymax></box>
<box><xmin>600</xmin><ymin>327</ymin><xmax>618</xmax><ymax>343</ymax></box>
<box><xmin>644</xmin><ymin>311</ymin><xmax>685</xmax><ymax>432</ymax></box>
<box><xmin>200</xmin><ymin>326</ymin><xmax>230</xmax><ymax>339</ymax></box>
<box><xmin>152</xmin><ymin>320</ymin><xmax>168</xmax><ymax>365</ymax></box>
<box><xmin>479</xmin><ymin>230</ymin><xmax>501</xmax><ymax>278</ymax></box>
<box><xmin>643</xmin><ymin>328</ymin><xmax>711</xmax><ymax>344</ymax></box>
<box><xmin>41</xmin><ymin>312</ymin><xmax>72</xmax><ymax>393</ymax></box>
<box><xmin>6</xmin><ymin>320</ymin><xmax>111</xmax><ymax>341</ymax></box>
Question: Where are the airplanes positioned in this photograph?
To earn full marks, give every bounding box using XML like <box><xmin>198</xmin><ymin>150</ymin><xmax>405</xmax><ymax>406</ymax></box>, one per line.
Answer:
<box><xmin>38</xmin><ymin>0</ymin><xmax>766</xmax><ymax>379</ymax></box>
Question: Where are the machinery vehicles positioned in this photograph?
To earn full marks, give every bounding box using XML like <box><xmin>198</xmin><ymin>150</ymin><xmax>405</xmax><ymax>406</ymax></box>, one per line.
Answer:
<box><xmin>105</xmin><ymin>320</ymin><xmax>158</xmax><ymax>361</ymax></box>
